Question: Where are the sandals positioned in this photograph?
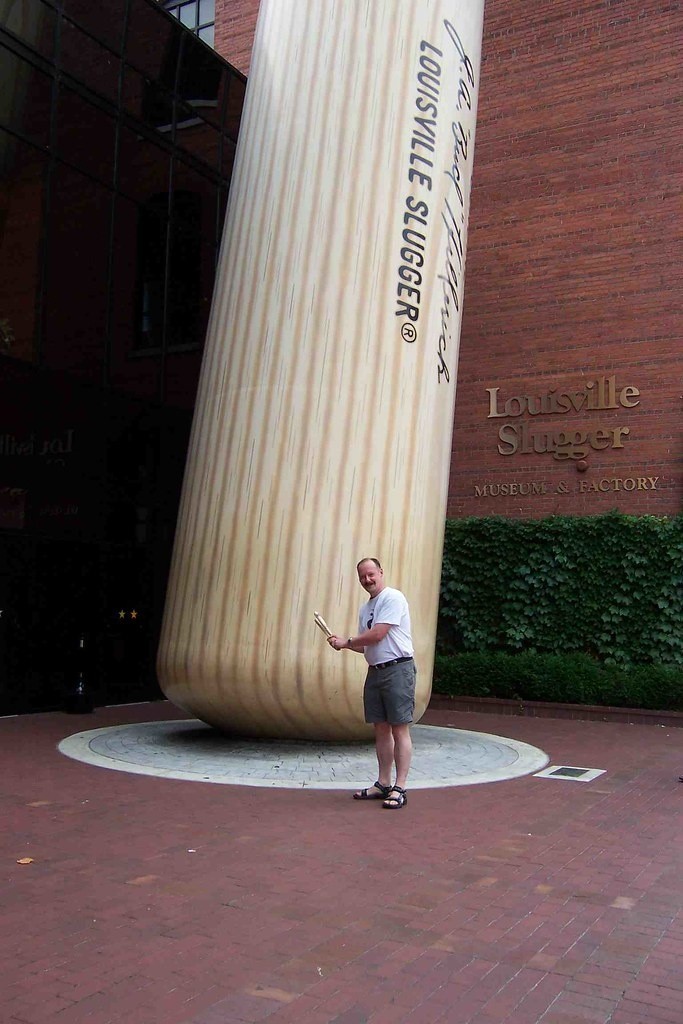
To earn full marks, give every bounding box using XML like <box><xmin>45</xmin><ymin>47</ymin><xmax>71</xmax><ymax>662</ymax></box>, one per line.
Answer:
<box><xmin>354</xmin><ymin>782</ymin><xmax>393</xmax><ymax>799</ymax></box>
<box><xmin>383</xmin><ymin>786</ymin><xmax>407</xmax><ymax>808</ymax></box>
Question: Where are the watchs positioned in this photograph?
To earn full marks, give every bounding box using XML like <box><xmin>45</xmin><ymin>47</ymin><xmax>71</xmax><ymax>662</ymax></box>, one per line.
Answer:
<box><xmin>348</xmin><ymin>636</ymin><xmax>352</xmax><ymax>647</ymax></box>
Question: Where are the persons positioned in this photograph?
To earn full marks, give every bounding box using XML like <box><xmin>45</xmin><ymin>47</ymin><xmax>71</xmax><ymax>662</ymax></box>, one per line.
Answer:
<box><xmin>327</xmin><ymin>557</ymin><xmax>418</xmax><ymax>809</ymax></box>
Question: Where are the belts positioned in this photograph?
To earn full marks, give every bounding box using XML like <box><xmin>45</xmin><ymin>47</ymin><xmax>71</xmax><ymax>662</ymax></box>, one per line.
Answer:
<box><xmin>369</xmin><ymin>657</ymin><xmax>413</xmax><ymax>669</ymax></box>
